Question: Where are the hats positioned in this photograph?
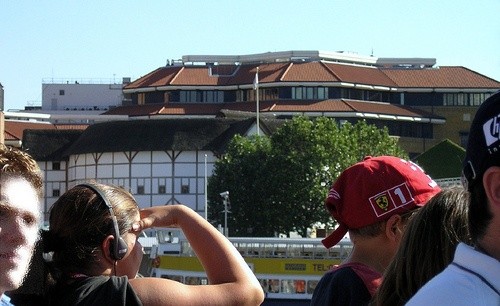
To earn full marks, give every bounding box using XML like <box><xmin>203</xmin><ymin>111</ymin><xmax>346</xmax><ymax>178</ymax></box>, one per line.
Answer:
<box><xmin>462</xmin><ymin>90</ymin><xmax>500</xmax><ymax>181</ymax></box>
<box><xmin>320</xmin><ymin>158</ymin><xmax>442</xmax><ymax>248</ymax></box>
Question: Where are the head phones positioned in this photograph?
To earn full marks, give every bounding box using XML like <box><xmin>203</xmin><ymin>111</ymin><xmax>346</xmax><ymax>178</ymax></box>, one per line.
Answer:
<box><xmin>74</xmin><ymin>183</ymin><xmax>127</xmax><ymax>261</ymax></box>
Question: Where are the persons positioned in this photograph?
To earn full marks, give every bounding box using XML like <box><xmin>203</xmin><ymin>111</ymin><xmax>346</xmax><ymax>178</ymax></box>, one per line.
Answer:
<box><xmin>405</xmin><ymin>88</ymin><xmax>500</xmax><ymax>306</ymax></box>
<box><xmin>30</xmin><ymin>182</ymin><xmax>265</xmax><ymax>306</ymax></box>
<box><xmin>376</xmin><ymin>186</ymin><xmax>473</xmax><ymax>306</ymax></box>
<box><xmin>309</xmin><ymin>155</ymin><xmax>443</xmax><ymax>306</ymax></box>
<box><xmin>0</xmin><ymin>142</ymin><xmax>47</xmax><ymax>306</ymax></box>
